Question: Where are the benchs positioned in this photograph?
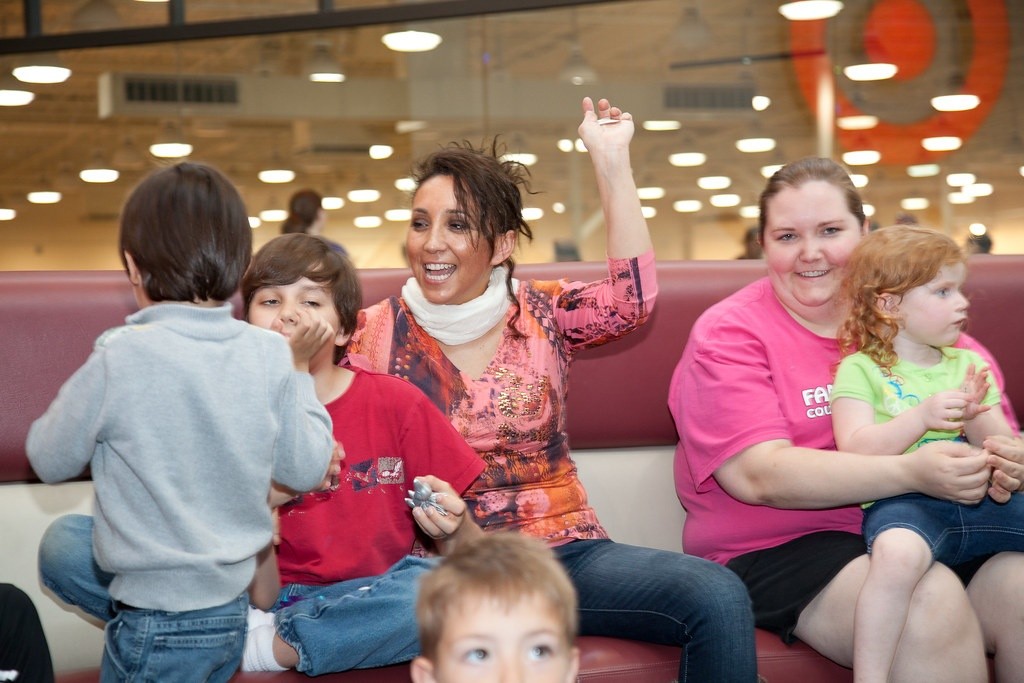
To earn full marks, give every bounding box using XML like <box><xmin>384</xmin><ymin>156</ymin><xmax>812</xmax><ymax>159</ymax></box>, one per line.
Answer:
<box><xmin>2</xmin><ymin>255</ymin><xmax>1024</xmax><ymax>683</ymax></box>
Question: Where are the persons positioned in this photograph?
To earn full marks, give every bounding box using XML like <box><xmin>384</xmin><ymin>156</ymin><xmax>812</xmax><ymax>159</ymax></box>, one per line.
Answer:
<box><xmin>668</xmin><ymin>155</ymin><xmax>1024</xmax><ymax>683</ymax></box>
<box><xmin>340</xmin><ymin>96</ymin><xmax>758</xmax><ymax>683</ymax></box>
<box><xmin>832</xmin><ymin>227</ymin><xmax>1024</xmax><ymax>683</ymax></box>
<box><xmin>282</xmin><ymin>190</ymin><xmax>349</xmax><ymax>269</ymax></box>
<box><xmin>38</xmin><ymin>234</ymin><xmax>488</xmax><ymax>677</ymax></box>
<box><xmin>738</xmin><ymin>228</ymin><xmax>764</xmax><ymax>261</ymax></box>
<box><xmin>410</xmin><ymin>536</ymin><xmax>581</xmax><ymax>683</ymax></box>
<box><xmin>25</xmin><ymin>163</ymin><xmax>333</xmax><ymax>683</ymax></box>
